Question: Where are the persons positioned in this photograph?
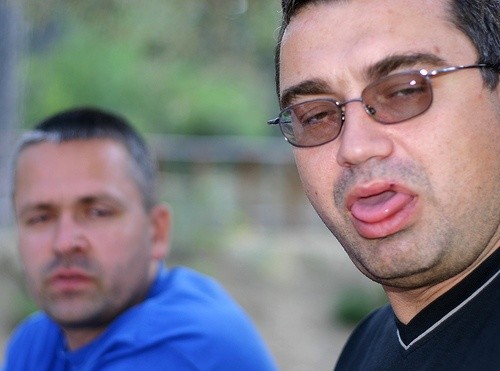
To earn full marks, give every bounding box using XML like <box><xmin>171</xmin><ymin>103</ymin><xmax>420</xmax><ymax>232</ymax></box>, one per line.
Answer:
<box><xmin>265</xmin><ymin>0</ymin><xmax>499</xmax><ymax>371</ymax></box>
<box><xmin>0</xmin><ymin>104</ymin><xmax>275</xmax><ymax>371</ymax></box>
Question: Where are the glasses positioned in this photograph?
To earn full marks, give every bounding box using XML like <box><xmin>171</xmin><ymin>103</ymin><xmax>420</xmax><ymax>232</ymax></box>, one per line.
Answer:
<box><xmin>266</xmin><ymin>64</ymin><xmax>495</xmax><ymax>147</ymax></box>
